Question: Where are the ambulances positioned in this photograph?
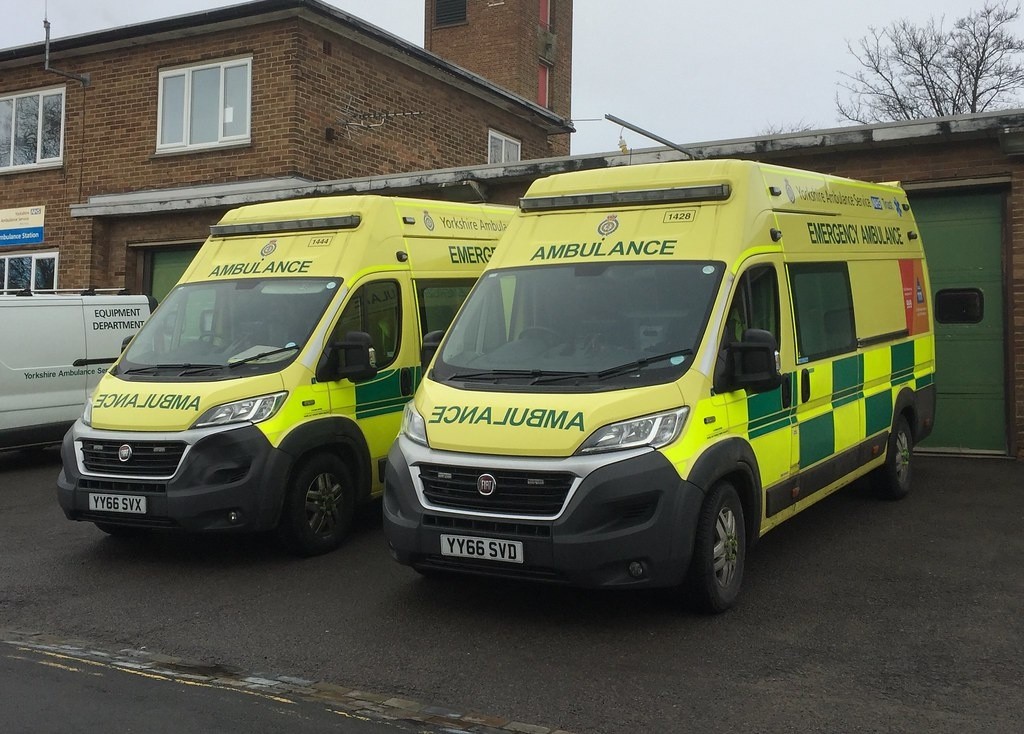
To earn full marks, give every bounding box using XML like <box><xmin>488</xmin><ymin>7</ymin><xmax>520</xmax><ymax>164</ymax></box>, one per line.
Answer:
<box><xmin>379</xmin><ymin>158</ymin><xmax>939</xmax><ymax>613</ymax></box>
<box><xmin>56</xmin><ymin>191</ymin><xmax>535</xmax><ymax>554</ymax></box>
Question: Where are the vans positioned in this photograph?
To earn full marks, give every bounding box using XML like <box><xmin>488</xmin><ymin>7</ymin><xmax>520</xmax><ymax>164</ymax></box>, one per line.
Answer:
<box><xmin>1</xmin><ymin>289</ymin><xmax>152</xmax><ymax>453</ymax></box>
